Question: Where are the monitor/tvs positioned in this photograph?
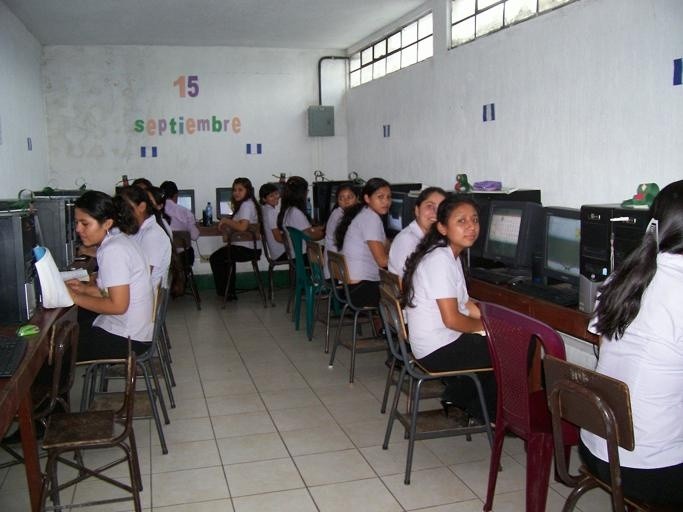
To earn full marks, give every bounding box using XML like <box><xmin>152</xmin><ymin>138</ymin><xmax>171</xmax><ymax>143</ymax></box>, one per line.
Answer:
<box><xmin>482</xmin><ymin>198</ymin><xmax>535</xmax><ymax>277</ymax></box>
<box><xmin>177</xmin><ymin>186</ymin><xmax>197</xmax><ymax>221</ymax></box>
<box><xmin>540</xmin><ymin>202</ymin><xmax>580</xmax><ymax>281</ymax></box>
<box><xmin>216</xmin><ymin>187</ymin><xmax>233</xmax><ymax>220</ymax></box>
<box><xmin>384</xmin><ymin>191</ymin><xmax>417</xmax><ymax>238</ymax></box>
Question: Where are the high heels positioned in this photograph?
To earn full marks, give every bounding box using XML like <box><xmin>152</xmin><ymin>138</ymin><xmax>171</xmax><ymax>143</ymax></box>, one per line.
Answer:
<box><xmin>440</xmin><ymin>398</ymin><xmax>470</xmax><ymax>417</ymax></box>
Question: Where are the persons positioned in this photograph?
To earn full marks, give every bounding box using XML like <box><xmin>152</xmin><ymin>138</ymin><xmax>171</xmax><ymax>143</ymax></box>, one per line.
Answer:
<box><xmin>258</xmin><ymin>182</ymin><xmax>287</xmax><ymax>262</ymax></box>
<box><xmin>76</xmin><ymin>185</ymin><xmax>172</xmax><ymax>337</ymax></box>
<box><xmin>384</xmin><ymin>186</ymin><xmax>446</xmax><ymax>371</ymax></box>
<box><xmin>132</xmin><ymin>177</ymin><xmax>151</xmax><ymax>190</ymax></box>
<box><xmin>321</xmin><ymin>184</ymin><xmax>360</xmax><ymax>285</ymax></box>
<box><xmin>397</xmin><ymin>195</ymin><xmax>497</xmax><ymax>429</ymax></box>
<box><xmin>277</xmin><ymin>176</ymin><xmax>328</xmax><ymax>300</ymax></box>
<box><xmin>334</xmin><ymin>177</ymin><xmax>395</xmax><ymax>338</ymax></box>
<box><xmin>577</xmin><ymin>180</ymin><xmax>683</xmax><ymax>512</ymax></box>
<box><xmin>209</xmin><ymin>178</ymin><xmax>262</xmax><ymax>303</ymax></box>
<box><xmin>159</xmin><ymin>181</ymin><xmax>200</xmax><ymax>299</ymax></box>
<box><xmin>1</xmin><ymin>189</ymin><xmax>155</xmax><ymax>446</ymax></box>
<box><xmin>143</xmin><ymin>186</ymin><xmax>175</xmax><ymax>243</ymax></box>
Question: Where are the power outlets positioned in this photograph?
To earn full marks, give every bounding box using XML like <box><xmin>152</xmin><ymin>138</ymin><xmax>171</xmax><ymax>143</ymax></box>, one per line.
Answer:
<box><xmin>200</xmin><ymin>256</ymin><xmax>211</xmax><ymax>263</ymax></box>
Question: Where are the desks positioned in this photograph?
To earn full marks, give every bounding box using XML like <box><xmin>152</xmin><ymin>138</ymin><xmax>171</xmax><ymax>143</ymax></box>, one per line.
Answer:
<box><xmin>0</xmin><ymin>254</ymin><xmax>96</xmax><ymax>512</ymax></box>
<box><xmin>193</xmin><ymin>220</ymin><xmax>225</xmax><ymax>237</ymax></box>
<box><xmin>464</xmin><ymin>271</ymin><xmax>601</xmax><ymax>391</ymax></box>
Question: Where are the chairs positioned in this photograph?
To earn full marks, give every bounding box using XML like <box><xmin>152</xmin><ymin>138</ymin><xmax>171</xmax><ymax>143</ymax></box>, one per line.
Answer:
<box><xmin>479</xmin><ymin>300</ymin><xmax>582</xmax><ymax>512</ymax></box>
<box><xmin>271</xmin><ymin>228</ymin><xmax>296</xmax><ymax>313</ymax></box>
<box><xmin>306</xmin><ymin>239</ymin><xmax>378</xmax><ymax>353</ymax></box>
<box><xmin>12</xmin><ymin>303</ymin><xmax>85</xmax><ymax>477</ymax></box>
<box><xmin>543</xmin><ymin>355</ymin><xmax>662</xmax><ymax>511</ymax></box>
<box><xmin>40</xmin><ymin>336</ymin><xmax>144</xmax><ymax>512</ymax></box>
<box><xmin>173</xmin><ymin>231</ymin><xmax>202</xmax><ymax>311</ymax></box>
<box><xmin>326</xmin><ymin>249</ymin><xmax>388</xmax><ymax>384</ymax></box>
<box><xmin>263</xmin><ymin>235</ymin><xmax>290</xmax><ymax>308</ymax></box>
<box><xmin>286</xmin><ymin>226</ymin><xmax>343</xmax><ymax>341</ymax></box>
<box><xmin>220</xmin><ymin>223</ymin><xmax>269</xmax><ymax>309</ymax></box>
<box><xmin>378</xmin><ymin>284</ymin><xmax>503</xmax><ymax>485</ymax></box>
<box><xmin>74</xmin><ymin>275</ymin><xmax>171</xmax><ymax>455</ymax></box>
<box><xmin>377</xmin><ymin>266</ymin><xmax>472</xmax><ymax>441</ymax></box>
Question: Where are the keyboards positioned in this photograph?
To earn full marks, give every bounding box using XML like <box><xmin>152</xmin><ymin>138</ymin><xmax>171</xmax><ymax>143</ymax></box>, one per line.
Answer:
<box><xmin>0</xmin><ymin>337</ymin><xmax>28</xmax><ymax>380</ymax></box>
<box><xmin>511</xmin><ymin>275</ymin><xmax>577</xmax><ymax>307</ymax></box>
<box><xmin>467</xmin><ymin>265</ymin><xmax>506</xmax><ymax>284</ymax></box>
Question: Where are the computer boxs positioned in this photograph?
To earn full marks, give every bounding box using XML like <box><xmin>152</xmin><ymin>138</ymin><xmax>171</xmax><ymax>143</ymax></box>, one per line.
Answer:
<box><xmin>613</xmin><ymin>208</ymin><xmax>649</xmax><ymax>272</ymax></box>
<box><xmin>23</xmin><ymin>197</ymin><xmax>77</xmax><ymax>267</ymax></box>
<box><xmin>2</xmin><ymin>206</ymin><xmax>37</xmax><ymax>324</ymax></box>
<box><xmin>580</xmin><ymin>204</ymin><xmax>614</xmax><ymax>314</ymax></box>
<box><xmin>313</xmin><ymin>181</ymin><xmax>357</xmax><ymax>226</ymax></box>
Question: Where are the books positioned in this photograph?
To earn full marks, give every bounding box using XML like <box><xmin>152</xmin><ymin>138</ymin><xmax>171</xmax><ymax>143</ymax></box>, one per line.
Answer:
<box><xmin>33</xmin><ymin>246</ymin><xmax>91</xmax><ymax>309</ymax></box>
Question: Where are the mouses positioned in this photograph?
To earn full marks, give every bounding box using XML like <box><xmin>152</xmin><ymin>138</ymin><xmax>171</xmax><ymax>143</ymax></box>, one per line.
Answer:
<box><xmin>19</xmin><ymin>323</ymin><xmax>42</xmax><ymax>336</ymax></box>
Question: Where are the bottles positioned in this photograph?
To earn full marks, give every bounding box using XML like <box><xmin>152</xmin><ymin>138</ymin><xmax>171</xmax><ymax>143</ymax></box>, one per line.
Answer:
<box><xmin>306</xmin><ymin>196</ymin><xmax>311</xmax><ymax>218</ymax></box>
<box><xmin>204</xmin><ymin>200</ymin><xmax>213</xmax><ymax>226</ymax></box>
